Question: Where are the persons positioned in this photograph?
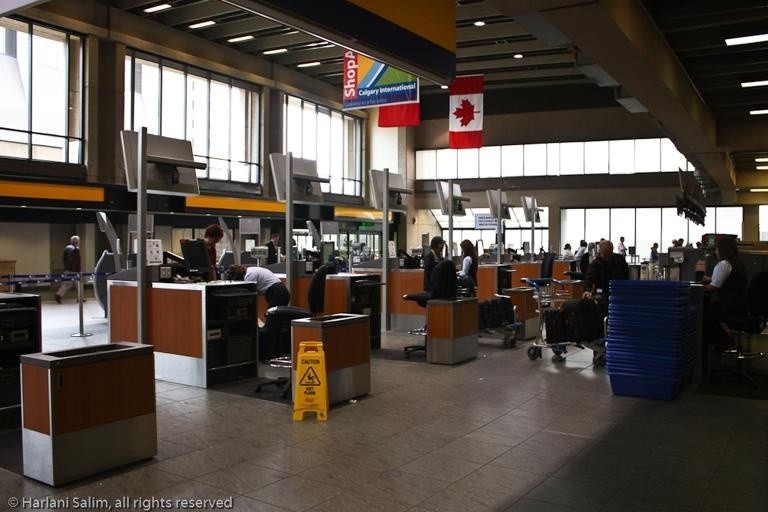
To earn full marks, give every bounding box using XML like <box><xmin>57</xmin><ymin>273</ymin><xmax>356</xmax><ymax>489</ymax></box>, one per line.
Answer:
<box><xmin>650</xmin><ymin>243</ymin><xmax>660</xmax><ymax>260</ymax></box>
<box><xmin>264</xmin><ymin>233</ymin><xmax>280</xmax><ymax>264</ymax></box>
<box><xmin>202</xmin><ymin>223</ymin><xmax>224</xmax><ymax>281</ymax></box>
<box><xmin>225</xmin><ymin>263</ymin><xmax>290</xmax><ymax>358</ymax></box>
<box><xmin>455</xmin><ymin>239</ymin><xmax>477</xmax><ymax>286</ymax></box>
<box><xmin>422</xmin><ymin>235</ymin><xmax>446</xmax><ymax>291</ymax></box>
<box><xmin>54</xmin><ymin>236</ymin><xmax>88</xmax><ymax>303</ymax></box>
<box><xmin>562</xmin><ymin>237</ymin><xmax>627</xmax><ymax>272</ymax></box>
<box><xmin>582</xmin><ymin>240</ymin><xmax>629</xmax><ymax>365</ymax></box>
<box><xmin>673</xmin><ymin>236</ymin><xmax>713</xmax><ymax>253</ymax></box>
<box><xmin>699</xmin><ymin>235</ymin><xmax>754</xmax><ymax>355</ymax></box>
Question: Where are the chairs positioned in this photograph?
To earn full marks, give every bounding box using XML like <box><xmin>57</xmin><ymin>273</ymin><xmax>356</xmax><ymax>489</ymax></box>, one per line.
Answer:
<box><xmin>707</xmin><ymin>271</ymin><xmax>767</xmax><ymax>388</ymax></box>
<box><xmin>563</xmin><ymin>252</ymin><xmax>589</xmax><ymax>277</ymax></box>
<box><xmin>255</xmin><ymin>264</ymin><xmax>330</xmax><ymax>399</ymax></box>
<box><xmin>520</xmin><ymin>251</ymin><xmax>556</xmax><ymax>283</ymax></box>
<box><xmin>401</xmin><ymin>259</ymin><xmax>457</xmax><ymax>360</ymax></box>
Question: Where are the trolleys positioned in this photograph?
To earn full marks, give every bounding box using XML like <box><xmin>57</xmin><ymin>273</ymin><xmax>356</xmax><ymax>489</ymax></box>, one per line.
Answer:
<box><xmin>518</xmin><ymin>276</ymin><xmax>607</xmax><ymax>372</ymax></box>
<box><xmin>453</xmin><ymin>271</ymin><xmax>523</xmax><ymax>352</ymax></box>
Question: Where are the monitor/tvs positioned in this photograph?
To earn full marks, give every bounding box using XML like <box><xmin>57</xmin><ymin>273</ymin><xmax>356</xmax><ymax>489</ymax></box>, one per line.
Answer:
<box><xmin>269</xmin><ymin>153</ymin><xmax>324</xmax><ymax>203</ymax></box>
<box><xmin>436</xmin><ymin>181</ymin><xmax>466</xmax><ymax>216</ymax></box>
<box><xmin>487</xmin><ymin>190</ymin><xmax>511</xmax><ymax>219</ymax></box>
<box><xmin>476</xmin><ymin>240</ymin><xmax>484</xmax><ymax>257</ymax></box>
<box><xmin>320</xmin><ymin>241</ymin><xmax>334</xmax><ymax>265</ymax></box>
<box><xmin>371</xmin><ymin>170</ymin><xmax>409</xmax><ymax>212</ymax></box>
<box><xmin>180</xmin><ymin>238</ymin><xmax>210</xmax><ymax>268</ymax></box>
<box><xmin>120</xmin><ymin>130</ymin><xmax>200</xmax><ymax>196</ymax></box>
<box><xmin>521</xmin><ymin>197</ymin><xmax>540</xmax><ymax>223</ymax></box>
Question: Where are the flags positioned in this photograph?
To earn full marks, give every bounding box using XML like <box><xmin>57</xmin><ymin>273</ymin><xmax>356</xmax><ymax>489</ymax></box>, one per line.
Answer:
<box><xmin>448</xmin><ymin>73</ymin><xmax>486</xmax><ymax>149</ymax></box>
<box><xmin>378</xmin><ymin>103</ymin><xmax>421</xmax><ymax>127</ymax></box>
<box><xmin>341</xmin><ymin>48</ymin><xmax>421</xmax><ymax>111</ymax></box>
